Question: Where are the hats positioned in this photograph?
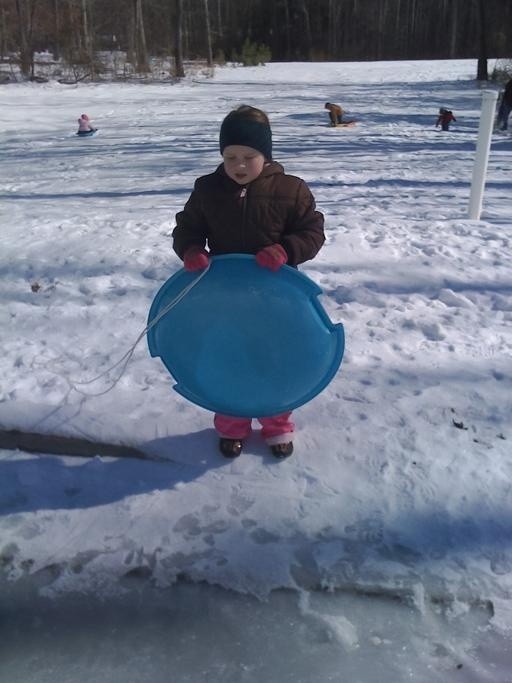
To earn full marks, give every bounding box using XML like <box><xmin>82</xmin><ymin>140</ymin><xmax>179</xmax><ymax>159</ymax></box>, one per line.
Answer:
<box><xmin>219</xmin><ymin>104</ymin><xmax>272</xmax><ymax>161</ymax></box>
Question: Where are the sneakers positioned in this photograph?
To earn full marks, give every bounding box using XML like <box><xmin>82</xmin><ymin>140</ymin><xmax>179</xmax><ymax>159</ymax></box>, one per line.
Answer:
<box><xmin>219</xmin><ymin>437</ymin><xmax>242</xmax><ymax>457</ymax></box>
<box><xmin>273</xmin><ymin>442</ymin><xmax>293</xmax><ymax>458</ymax></box>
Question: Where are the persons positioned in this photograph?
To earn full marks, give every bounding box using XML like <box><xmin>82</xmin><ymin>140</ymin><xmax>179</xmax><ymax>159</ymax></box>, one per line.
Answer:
<box><xmin>171</xmin><ymin>104</ymin><xmax>325</xmax><ymax>459</ymax></box>
<box><xmin>324</xmin><ymin>101</ymin><xmax>345</xmax><ymax>126</ymax></box>
<box><xmin>435</xmin><ymin>106</ymin><xmax>456</xmax><ymax>131</ymax></box>
<box><xmin>493</xmin><ymin>78</ymin><xmax>511</xmax><ymax>131</ymax></box>
<box><xmin>76</xmin><ymin>114</ymin><xmax>94</xmax><ymax>133</ymax></box>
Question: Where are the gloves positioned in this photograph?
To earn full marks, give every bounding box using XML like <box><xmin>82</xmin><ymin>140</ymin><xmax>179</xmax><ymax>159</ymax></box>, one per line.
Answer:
<box><xmin>255</xmin><ymin>244</ymin><xmax>287</xmax><ymax>272</ymax></box>
<box><xmin>182</xmin><ymin>244</ymin><xmax>210</xmax><ymax>271</ymax></box>
